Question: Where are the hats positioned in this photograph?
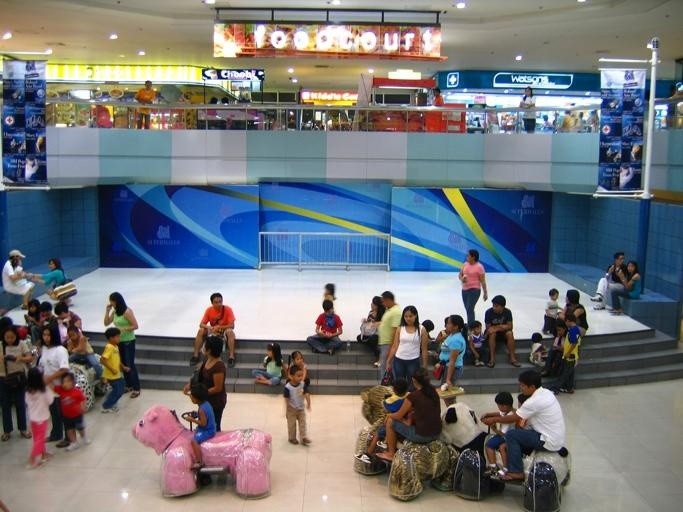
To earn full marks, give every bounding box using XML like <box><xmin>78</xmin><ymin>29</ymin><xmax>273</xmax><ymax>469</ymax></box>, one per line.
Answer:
<box><xmin>9</xmin><ymin>250</ymin><xmax>26</xmax><ymax>258</ymax></box>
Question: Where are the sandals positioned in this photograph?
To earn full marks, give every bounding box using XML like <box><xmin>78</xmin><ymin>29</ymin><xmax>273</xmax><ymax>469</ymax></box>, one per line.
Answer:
<box><xmin>131</xmin><ymin>392</ymin><xmax>140</xmax><ymax>398</ymax></box>
<box><xmin>500</xmin><ymin>473</ymin><xmax>525</xmax><ymax>481</ymax></box>
<box><xmin>492</xmin><ymin>468</ymin><xmax>507</xmax><ymax>479</ymax></box>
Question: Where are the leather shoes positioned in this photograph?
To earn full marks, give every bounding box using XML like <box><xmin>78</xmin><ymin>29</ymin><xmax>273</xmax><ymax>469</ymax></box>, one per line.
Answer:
<box><xmin>610</xmin><ymin>308</ymin><xmax>623</xmax><ymax>312</ymax></box>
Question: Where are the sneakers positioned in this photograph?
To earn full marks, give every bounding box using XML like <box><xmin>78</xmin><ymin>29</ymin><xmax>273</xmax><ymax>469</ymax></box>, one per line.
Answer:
<box><xmin>301</xmin><ymin>438</ymin><xmax>310</xmax><ymax>443</ymax></box>
<box><xmin>109</xmin><ymin>406</ymin><xmax>120</xmax><ymax>413</ymax></box>
<box><xmin>2</xmin><ymin>434</ymin><xmax>11</xmax><ymax>441</ymax></box>
<box><xmin>482</xmin><ymin>467</ymin><xmax>496</xmax><ymax>476</ymax></box>
<box><xmin>191</xmin><ymin>356</ymin><xmax>197</xmax><ymax>365</ymax></box>
<box><xmin>227</xmin><ymin>359</ymin><xmax>233</xmax><ymax>366</ymax></box>
<box><xmin>289</xmin><ymin>438</ymin><xmax>298</xmax><ymax>443</ymax></box>
<box><xmin>590</xmin><ymin>294</ymin><xmax>602</xmax><ymax>301</ymax></box>
<box><xmin>377</xmin><ymin>440</ymin><xmax>388</xmax><ymax>449</ymax></box>
<box><xmin>352</xmin><ymin>452</ymin><xmax>373</xmax><ymax>464</ymax></box>
<box><xmin>191</xmin><ymin>461</ymin><xmax>205</xmax><ymax>469</ymax></box>
<box><xmin>594</xmin><ymin>303</ymin><xmax>604</xmax><ymax>309</ymax></box>
<box><xmin>21</xmin><ymin>431</ymin><xmax>32</xmax><ymax>438</ymax></box>
<box><xmin>68</xmin><ymin>443</ymin><xmax>79</xmax><ymax>449</ymax></box>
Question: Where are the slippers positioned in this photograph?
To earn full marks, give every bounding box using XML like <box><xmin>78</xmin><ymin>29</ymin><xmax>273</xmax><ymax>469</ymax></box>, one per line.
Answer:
<box><xmin>511</xmin><ymin>359</ymin><xmax>521</xmax><ymax>367</ymax></box>
<box><xmin>374</xmin><ymin>452</ymin><xmax>394</xmax><ymax>463</ymax></box>
<box><xmin>486</xmin><ymin>360</ymin><xmax>494</xmax><ymax>367</ymax></box>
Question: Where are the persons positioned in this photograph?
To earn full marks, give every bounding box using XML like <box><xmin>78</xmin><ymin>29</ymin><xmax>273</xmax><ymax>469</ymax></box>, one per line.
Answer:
<box><xmin>324</xmin><ymin>282</ymin><xmax>337</xmax><ymax>305</ymax></box>
<box><xmin>133</xmin><ymin>80</ymin><xmax>158</xmax><ymax>131</ymax></box>
<box><xmin>91</xmin><ymin>99</ymin><xmax>114</xmax><ymax>129</ymax></box>
<box><xmin>619</xmin><ymin>162</ymin><xmax>634</xmax><ymax>190</ymax></box>
<box><xmin>499</xmin><ymin>112</ymin><xmax>517</xmax><ymax>132</ymax></box>
<box><xmin>0</xmin><ymin>250</ymin><xmax>36</xmax><ymax>311</ymax></box>
<box><xmin>459</xmin><ymin>249</ymin><xmax>488</xmax><ymax>324</ymax></box>
<box><xmin>541</xmin><ymin>103</ymin><xmax>599</xmax><ymax>133</ymax></box>
<box><xmin>518</xmin><ymin>86</ymin><xmax>537</xmax><ymax>133</ymax></box>
<box><xmin>188</xmin><ymin>382</ymin><xmax>219</xmax><ymax>470</ymax></box>
<box><xmin>0</xmin><ymin>292</ymin><xmax>142</xmax><ymax>471</ymax></box>
<box><xmin>189</xmin><ymin>291</ymin><xmax>238</xmax><ymax>367</ymax></box>
<box><xmin>218</xmin><ymin>97</ymin><xmax>236</xmax><ymax>118</ymax></box>
<box><xmin>610</xmin><ymin>260</ymin><xmax>642</xmax><ymax>313</ymax></box>
<box><xmin>251</xmin><ymin>343</ymin><xmax>289</xmax><ymax>386</ymax></box>
<box><xmin>356</xmin><ymin>287</ymin><xmax>588</xmax><ymax>479</ymax></box>
<box><xmin>284</xmin><ymin>351</ymin><xmax>309</xmax><ymax>383</ymax></box>
<box><xmin>307</xmin><ymin>300</ymin><xmax>343</xmax><ymax>356</ymax></box>
<box><xmin>665</xmin><ymin>80</ymin><xmax>683</xmax><ymax>128</ymax></box>
<box><xmin>205</xmin><ymin>97</ymin><xmax>219</xmax><ymax>115</ymax></box>
<box><xmin>181</xmin><ymin>335</ymin><xmax>229</xmax><ymax>431</ymax></box>
<box><xmin>26</xmin><ymin>257</ymin><xmax>75</xmax><ymax>311</ymax></box>
<box><xmin>590</xmin><ymin>252</ymin><xmax>631</xmax><ymax>310</ymax></box>
<box><xmin>282</xmin><ymin>365</ymin><xmax>314</xmax><ymax>446</ymax></box>
<box><xmin>25</xmin><ymin>153</ymin><xmax>39</xmax><ymax>181</ymax></box>
<box><xmin>429</xmin><ymin>87</ymin><xmax>446</xmax><ymax>132</ymax></box>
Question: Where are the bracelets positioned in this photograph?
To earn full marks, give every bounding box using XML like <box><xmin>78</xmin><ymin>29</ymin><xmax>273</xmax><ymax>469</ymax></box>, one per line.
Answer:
<box><xmin>605</xmin><ymin>273</ymin><xmax>610</xmax><ymax>278</ymax></box>
<box><xmin>621</xmin><ymin>278</ymin><xmax>627</xmax><ymax>281</ymax></box>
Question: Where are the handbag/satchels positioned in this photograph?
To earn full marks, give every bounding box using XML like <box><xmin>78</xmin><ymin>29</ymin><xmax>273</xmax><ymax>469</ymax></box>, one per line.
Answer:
<box><xmin>53</xmin><ymin>278</ymin><xmax>77</xmax><ymax>300</ymax></box>
<box><xmin>381</xmin><ymin>370</ymin><xmax>395</xmax><ymax>387</ymax></box>
<box><xmin>5</xmin><ymin>371</ymin><xmax>26</xmax><ymax>387</ymax></box>
<box><xmin>433</xmin><ymin>360</ymin><xmax>444</xmax><ymax>378</ymax></box>
